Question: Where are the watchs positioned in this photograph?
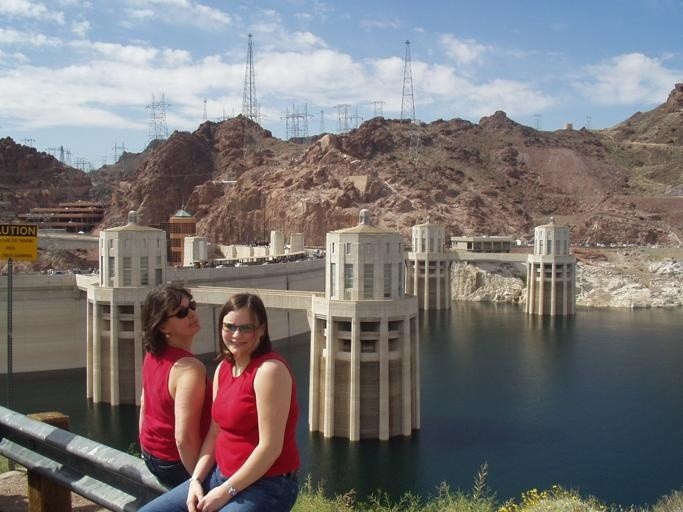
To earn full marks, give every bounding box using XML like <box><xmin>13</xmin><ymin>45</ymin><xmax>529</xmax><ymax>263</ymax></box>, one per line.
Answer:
<box><xmin>223</xmin><ymin>480</ymin><xmax>238</xmax><ymax>497</ymax></box>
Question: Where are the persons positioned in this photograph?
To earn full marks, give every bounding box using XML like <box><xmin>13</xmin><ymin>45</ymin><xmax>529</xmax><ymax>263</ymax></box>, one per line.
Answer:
<box><xmin>135</xmin><ymin>282</ymin><xmax>214</xmax><ymax>485</ymax></box>
<box><xmin>136</xmin><ymin>293</ymin><xmax>301</xmax><ymax>512</ymax></box>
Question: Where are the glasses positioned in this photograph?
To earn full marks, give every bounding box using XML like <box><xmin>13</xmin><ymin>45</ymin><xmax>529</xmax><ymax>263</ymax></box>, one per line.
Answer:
<box><xmin>164</xmin><ymin>300</ymin><xmax>197</xmax><ymax>319</ymax></box>
<box><xmin>221</xmin><ymin>321</ymin><xmax>263</xmax><ymax>333</ymax></box>
